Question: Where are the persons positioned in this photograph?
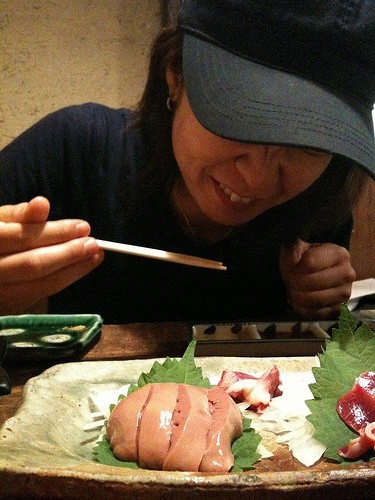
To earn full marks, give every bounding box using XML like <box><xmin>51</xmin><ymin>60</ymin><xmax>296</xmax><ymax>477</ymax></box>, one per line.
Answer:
<box><xmin>0</xmin><ymin>0</ymin><xmax>375</xmax><ymax>320</ymax></box>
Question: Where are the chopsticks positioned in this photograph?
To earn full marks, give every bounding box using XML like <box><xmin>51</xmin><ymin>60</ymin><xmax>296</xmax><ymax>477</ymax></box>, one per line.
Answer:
<box><xmin>94</xmin><ymin>239</ymin><xmax>228</xmax><ymax>271</ymax></box>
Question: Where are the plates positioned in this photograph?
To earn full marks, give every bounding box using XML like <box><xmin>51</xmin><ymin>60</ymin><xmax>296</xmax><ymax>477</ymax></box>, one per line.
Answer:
<box><xmin>0</xmin><ymin>357</ymin><xmax>375</xmax><ymax>500</ymax></box>
<box><xmin>0</xmin><ymin>314</ymin><xmax>104</xmax><ymax>362</ymax></box>
<box><xmin>191</xmin><ymin>321</ymin><xmax>339</xmax><ymax>356</ymax></box>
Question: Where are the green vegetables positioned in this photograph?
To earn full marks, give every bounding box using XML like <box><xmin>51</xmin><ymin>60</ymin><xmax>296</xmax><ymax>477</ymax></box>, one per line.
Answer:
<box><xmin>305</xmin><ymin>302</ymin><xmax>375</xmax><ymax>463</ymax></box>
<box><xmin>92</xmin><ymin>335</ymin><xmax>262</xmax><ymax>473</ymax></box>
